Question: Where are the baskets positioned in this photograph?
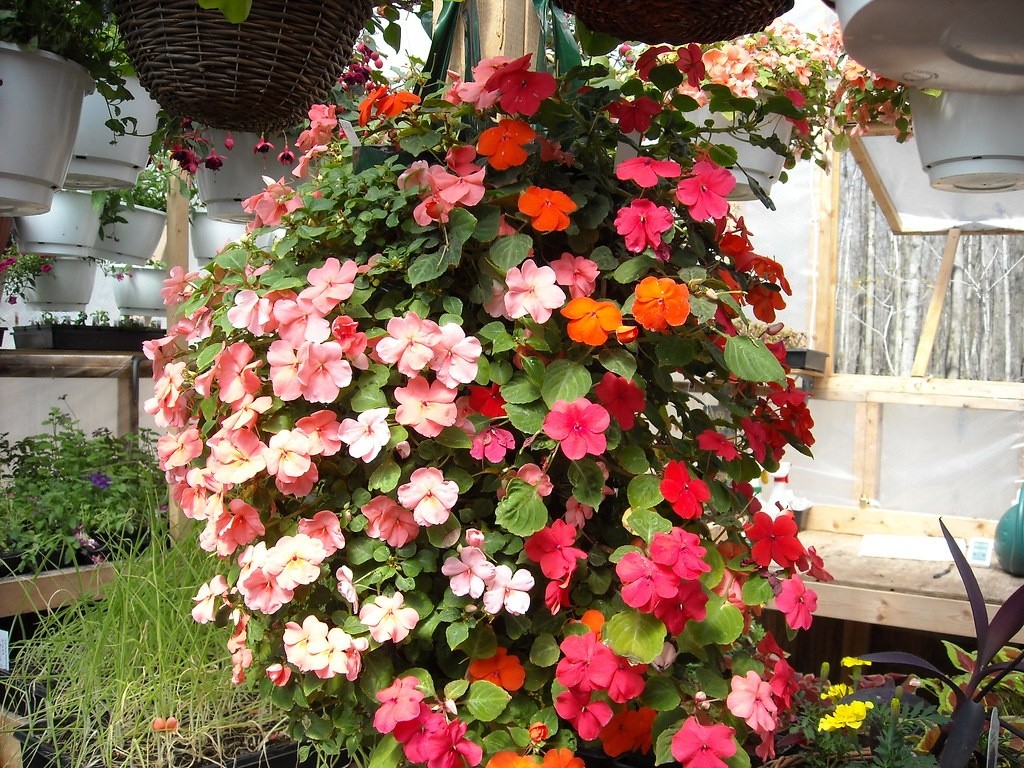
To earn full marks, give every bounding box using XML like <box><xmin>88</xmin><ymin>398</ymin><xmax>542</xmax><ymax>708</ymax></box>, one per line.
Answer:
<box><xmin>552</xmin><ymin>0</ymin><xmax>795</xmax><ymax>47</ymax></box>
<box><xmin>111</xmin><ymin>0</ymin><xmax>372</xmax><ymax>135</ymax></box>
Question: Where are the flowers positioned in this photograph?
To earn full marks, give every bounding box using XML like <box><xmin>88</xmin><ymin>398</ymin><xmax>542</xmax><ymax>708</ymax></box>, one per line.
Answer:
<box><xmin>0</xmin><ymin>19</ymin><xmax>928</xmax><ymax>768</ymax></box>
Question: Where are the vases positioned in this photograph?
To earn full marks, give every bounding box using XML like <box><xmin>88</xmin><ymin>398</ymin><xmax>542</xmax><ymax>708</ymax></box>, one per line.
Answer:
<box><xmin>19</xmin><ymin>252</ymin><xmax>98</xmax><ymax>312</ymax></box>
<box><xmin>0</xmin><ymin>35</ymin><xmax>91</xmax><ymax>219</ymax></box>
<box><xmin>15</xmin><ymin>188</ymin><xmax>109</xmax><ymax>257</ymax></box>
<box><xmin>828</xmin><ymin>0</ymin><xmax>1024</xmax><ymax>94</ymax></box>
<box><xmin>781</xmin><ymin>347</ymin><xmax>830</xmax><ymax>373</ymax></box>
<box><xmin>905</xmin><ymin>89</ymin><xmax>1024</xmax><ymax>195</ymax></box>
<box><xmin>677</xmin><ymin>92</ymin><xmax>795</xmax><ymax>202</ymax></box>
<box><xmin>190</xmin><ymin>208</ymin><xmax>289</xmax><ymax>272</ymax></box>
<box><xmin>14</xmin><ymin>710</ymin><xmax>348</xmax><ymax>768</ymax></box>
<box><xmin>12</xmin><ymin>323</ymin><xmax>168</xmax><ymax>354</ymax></box>
<box><xmin>111</xmin><ymin>262</ymin><xmax>170</xmax><ymax>320</ymax></box>
<box><xmin>93</xmin><ymin>193</ymin><xmax>169</xmax><ymax>268</ymax></box>
<box><xmin>59</xmin><ymin>51</ymin><xmax>163</xmax><ymax>190</ymax></box>
<box><xmin>186</xmin><ymin>119</ymin><xmax>331</xmax><ymax>205</ymax></box>
